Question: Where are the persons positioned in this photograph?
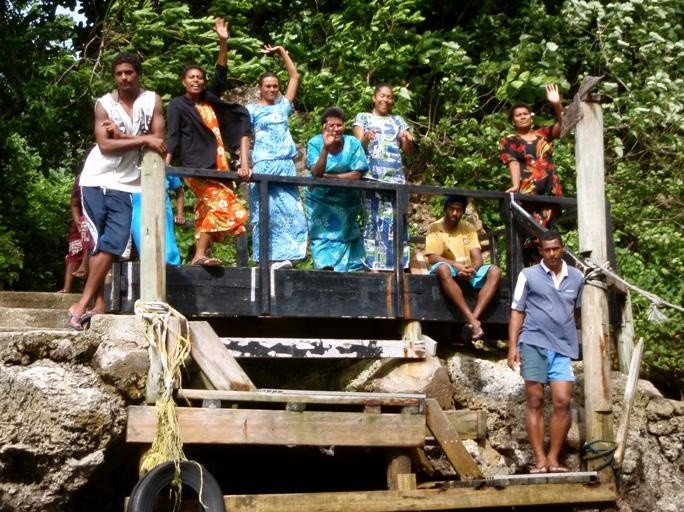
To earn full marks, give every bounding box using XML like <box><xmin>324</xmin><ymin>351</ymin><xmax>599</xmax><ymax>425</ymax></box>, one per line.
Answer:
<box><xmin>424</xmin><ymin>193</ymin><xmax>501</xmax><ymax>340</ymax></box>
<box><xmin>57</xmin><ymin>145</ymin><xmax>95</xmax><ymax>293</ymax></box>
<box><xmin>129</xmin><ymin>166</ymin><xmax>185</xmax><ymax>267</ymax></box>
<box><xmin>506</xmin><ymin>230</ymin><xmax>586</xmax><ymax>473</ymax></box>
<box><xmin>500</xmin><ymin>82</ymin><xmax>565</xmax><ymax>266</ymax></box>
<box><xmin>353</xmin><ymin>84</ymin><xmax>417</xmax><ymax>272</ymax></box>
<box><xmin>67</xmin><ymin>52</ymin><xmax>168</xmax><ymax>330</ymax></box>
<box><xmin>237</xmin><ymin>43</ymin><xmax>308</xmax><ymax>270</ymax></box>
<box><xmin>304</xmin><ymin>107</ymin><xmax>369</xmax><ymax>273</ymax></box>
<box><xmin>162</xmin><ymin>18</ymin><xmax>252</xmax><ymax>265</ymax></box>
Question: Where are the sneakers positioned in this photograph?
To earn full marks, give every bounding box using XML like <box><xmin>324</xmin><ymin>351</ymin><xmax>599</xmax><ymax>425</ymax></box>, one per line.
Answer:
<box><xmin>273</xmin><ymin>259</ymin><xmax>293</xmax><ymax>269</ymax></box>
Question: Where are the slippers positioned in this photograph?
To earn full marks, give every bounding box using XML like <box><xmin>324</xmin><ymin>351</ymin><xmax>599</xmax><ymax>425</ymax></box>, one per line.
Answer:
<box><xmin>73</xmin><ymin>270</ymin><xmax>88</xmax><ymax>279</ymax></box>
<box><xmin>530</xmin><ymin>464</ymin><xmax>547</xmax><ymax>473</ymax></box>
<box><xmin>80</xmin><ymin>311</ymin><xmax>94</xmax><ymax>323</ymax></box>
<box><xmin>548</xmin><ymin>464</ymin><xmax>569</xmax><ymax>472</ymax></box>
<box><xmin>59</xmin><ymin>288</ymin><xmax>70</xmax><ymax>293</ymax></box>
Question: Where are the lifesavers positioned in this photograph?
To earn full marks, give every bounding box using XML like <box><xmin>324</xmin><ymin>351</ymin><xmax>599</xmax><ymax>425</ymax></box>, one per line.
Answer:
<box><xmin>127</xmin><ymin>460</ymin><xmax>225</xmax><ymax>512</ymax></box>
